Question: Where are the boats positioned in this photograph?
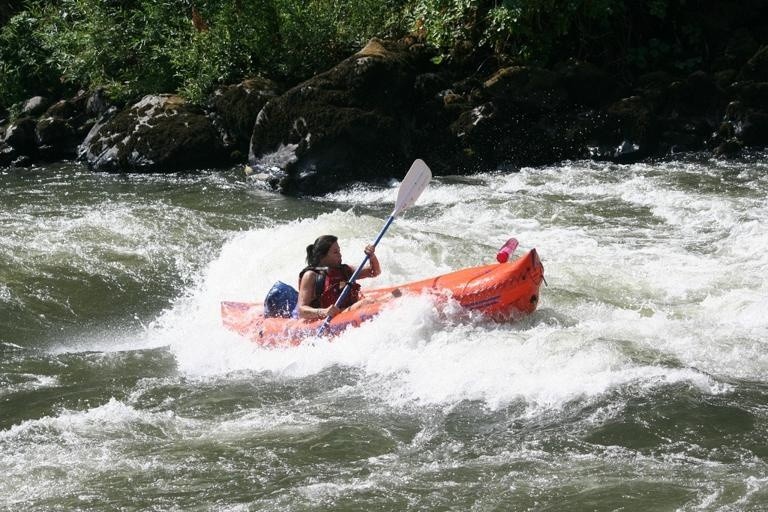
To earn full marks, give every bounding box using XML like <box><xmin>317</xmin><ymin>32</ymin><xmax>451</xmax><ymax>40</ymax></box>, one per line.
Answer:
<box><xmin>215</xmin><ymin>247</ymin><xmax>545</xmax><ymax>351</ymax></box>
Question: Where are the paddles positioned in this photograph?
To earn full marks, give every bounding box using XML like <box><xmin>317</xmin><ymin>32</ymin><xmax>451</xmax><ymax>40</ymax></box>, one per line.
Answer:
<box><xmin>309</xmin><ymin>159</ymin><xmax>432</xmax><ymax>351</ymax></box>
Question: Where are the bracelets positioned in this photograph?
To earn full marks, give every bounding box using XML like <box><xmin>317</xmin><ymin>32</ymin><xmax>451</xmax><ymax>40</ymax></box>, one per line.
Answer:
<box><xmin>316</xmin><ymin>308</ymin><xmax>324</xmax><ymax>318</ymax></box>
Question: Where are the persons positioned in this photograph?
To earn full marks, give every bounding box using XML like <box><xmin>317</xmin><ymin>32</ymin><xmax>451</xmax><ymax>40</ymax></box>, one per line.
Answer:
<box><xmin>295</xmin><ymin>235</ymin><xmax>380</xmax><ymax>320</ymax></box>
